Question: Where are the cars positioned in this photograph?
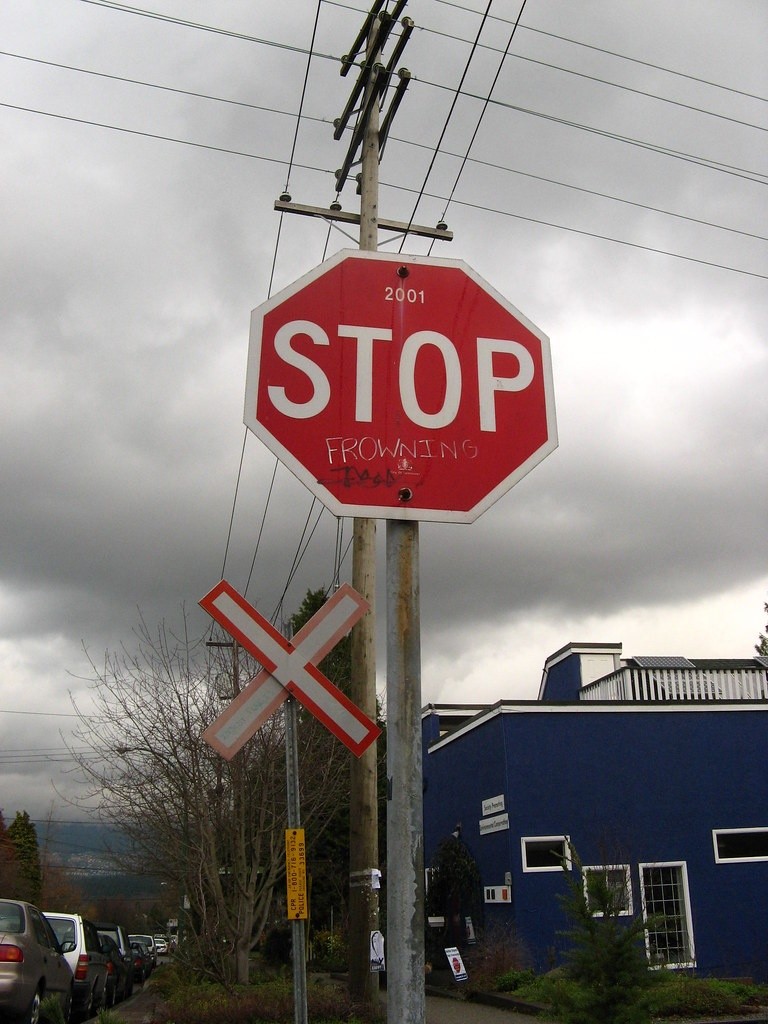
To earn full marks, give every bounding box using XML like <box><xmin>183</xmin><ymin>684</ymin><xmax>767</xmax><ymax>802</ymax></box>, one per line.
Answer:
<box><xmin>41</xmin><ymin>911</ymin><xmax>183</xmax><ymax>1020</ymax></box>
<box><xmin>0</xmin><ymin>898</ymin><xmax>77</xmax><ymax>1024</ymax></box>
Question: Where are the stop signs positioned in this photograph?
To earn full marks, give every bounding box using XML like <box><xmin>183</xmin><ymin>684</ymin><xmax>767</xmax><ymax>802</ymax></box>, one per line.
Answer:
<box><xmin>243</xmin><ymin>248</ymin><xmax>559</xmax><ymax>524</ymax></box>
